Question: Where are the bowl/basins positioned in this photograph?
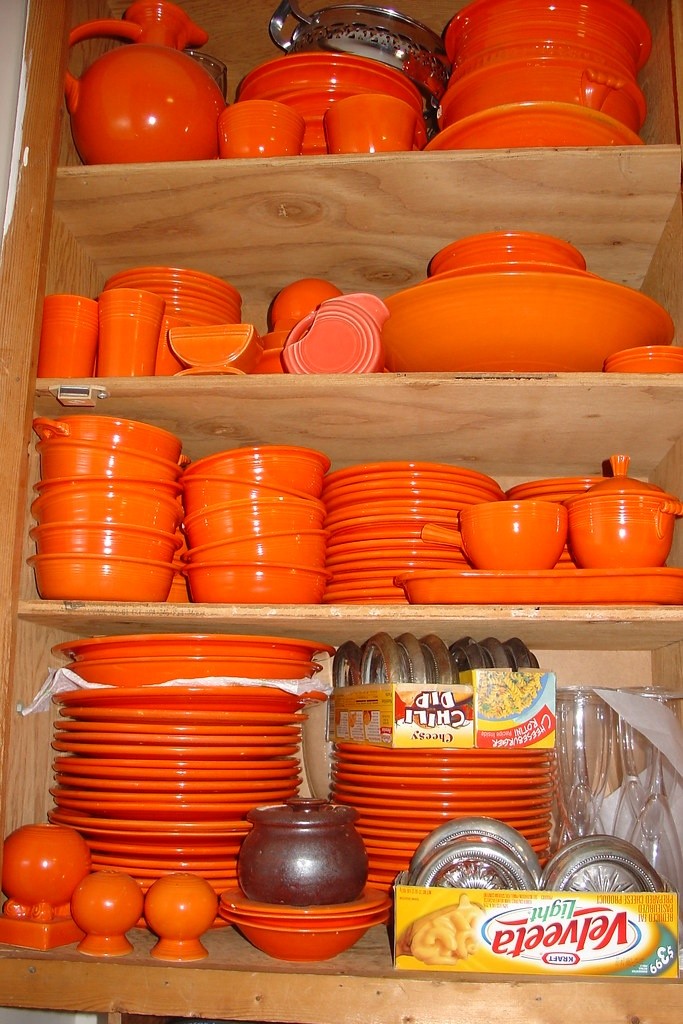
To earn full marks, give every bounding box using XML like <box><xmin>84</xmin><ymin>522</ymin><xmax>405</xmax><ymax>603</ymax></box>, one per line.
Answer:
<box><xmin>560</xmin><ymin>454</ymin><xmax>683</xmax><ymax>570</ymax></box>
<box><xmin>380</xmin><ymin>232</ymin><xmax>674</xmax><ymax>376</ymax></box>
<box><xmin>24</xmin><ymin>412</ymin><xmax>331</xmax><ymax>607</ymax></box>
<box><xmin>420</xmin><ymin>500</ymin><xmax>568</xmax><ymax>573</ymax></box>
<box><xmin>235</xmin><ymin>1</ymin><xmax>653</xmax><ymax>151</ymax></box>
<box><xmin>219</xmin><ymin>901</ymin><xmax>393</xmax><ymax>963</ymax></box>
<box><xmin>105</xmin><ymin>266</ymin><xmax>243</xmax><ymax>376</ymax></box>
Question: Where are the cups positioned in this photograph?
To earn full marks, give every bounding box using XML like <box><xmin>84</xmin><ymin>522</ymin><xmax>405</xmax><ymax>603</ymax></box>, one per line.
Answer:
<box><xmin>322</xmin><ymin>93</ymin><xmax>418</xmax><ymax>154</ymax></box>
<box><xmin>37</xmin><ymin>295</ymin><xmax>98</xmax><ymax>378</ymax></box>
<box><xmin>95</xmin><ymin>288</ymin><xmax>166</xmax><ymax>377</ymax></box>
<box><xmin>553</xmin><ymin>686</ymin><xmax>683</xmax><ymax>894</ymax></box>
<box><xmin>217</xmin><ymin>100</ymin><xmax>307</xmax><ymax>159</ymax></box>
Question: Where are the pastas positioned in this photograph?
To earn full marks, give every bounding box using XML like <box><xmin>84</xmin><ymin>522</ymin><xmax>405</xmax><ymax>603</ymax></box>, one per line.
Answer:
<box><xmin>476</xmin><ymin>671</ymin><xmax>545</xmax><ymax>719</ymax></box>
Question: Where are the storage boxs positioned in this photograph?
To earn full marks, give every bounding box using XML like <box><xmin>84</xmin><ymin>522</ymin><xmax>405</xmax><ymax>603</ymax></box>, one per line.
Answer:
<box><xmin>460</xmin><ymin>667</ymin><xmax>557</xmax><ymax>749</ymax></box>
<box><xmin>326</xmin><ymin>680</ymin><xmax>474</xmax><ymax>749</ymax></box>
<box><xmin>390</xmin><ymin>870</ymin><xmax>680</xmax><ymax>980</ymax></box>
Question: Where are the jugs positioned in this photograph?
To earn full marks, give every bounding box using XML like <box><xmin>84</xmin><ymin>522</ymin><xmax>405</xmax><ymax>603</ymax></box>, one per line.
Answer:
<box><xmin>65</xmin><ymin>0</ymin><xmax>227</xmax><ymax>164</ymax></box>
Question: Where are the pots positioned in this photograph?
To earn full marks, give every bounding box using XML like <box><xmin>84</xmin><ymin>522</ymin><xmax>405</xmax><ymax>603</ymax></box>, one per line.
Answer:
<box><xmin>268</xmin><ymin>0</ymin><xmax>450</xmax><ymax>123</ymax></box>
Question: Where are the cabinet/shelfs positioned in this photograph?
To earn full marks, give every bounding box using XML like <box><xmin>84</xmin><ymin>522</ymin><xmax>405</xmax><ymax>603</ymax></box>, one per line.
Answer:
<box><xmin>0</xmin><ymin>0</ymin><xmax>683</xmax><ymax>1024</ymax></box>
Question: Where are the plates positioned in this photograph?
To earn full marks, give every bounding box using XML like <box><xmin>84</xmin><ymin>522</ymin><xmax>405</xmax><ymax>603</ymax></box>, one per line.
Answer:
<box><xmin>44</xmin><ymin>628</ymin><xmax>560</xmax><ymax>916</ymax></box>
<box><xmin>604</xmin><ymin>344</ymin><xmax>683</xmax><ymax>373</ymax></box>
<box><xmin>315</xmin><ymin>462</ymin><xmax>609</xmax><ymax>604</ymax></box>
